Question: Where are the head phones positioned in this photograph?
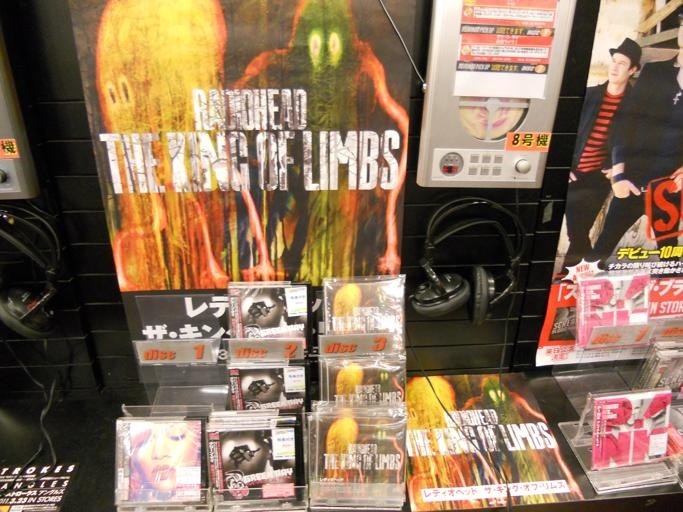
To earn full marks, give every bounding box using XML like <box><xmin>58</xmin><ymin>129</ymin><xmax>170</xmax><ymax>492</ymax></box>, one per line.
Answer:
<box><xmin>1</xmin><ymin>205</ymin><xmax>73</xmax><ymax>340</ymax></box>
<box><xmin>410</xmin><ymin>197</ymin><xmax>522</xmax><ymax>336</ymax></box>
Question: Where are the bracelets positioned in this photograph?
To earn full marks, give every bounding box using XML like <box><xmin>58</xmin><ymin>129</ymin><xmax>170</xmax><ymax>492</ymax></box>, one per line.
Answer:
<box><xmin>610</xmin><ymin>172</ymin><xmax>625</xmax><ymax>184</ymax></box>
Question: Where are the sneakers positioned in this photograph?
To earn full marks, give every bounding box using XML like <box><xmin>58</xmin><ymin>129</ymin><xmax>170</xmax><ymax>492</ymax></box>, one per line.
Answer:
<box><xmin>560</xmin><ymin>231</ymin><xmax>591</xmax><ymax>266</ymax></box>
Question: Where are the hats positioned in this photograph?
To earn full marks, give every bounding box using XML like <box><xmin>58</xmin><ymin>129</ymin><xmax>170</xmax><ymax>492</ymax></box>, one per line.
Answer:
<box><xmin>609</xmin><ymin>37</ymin><xmax>640</xmax><ymax>70</ymax></box>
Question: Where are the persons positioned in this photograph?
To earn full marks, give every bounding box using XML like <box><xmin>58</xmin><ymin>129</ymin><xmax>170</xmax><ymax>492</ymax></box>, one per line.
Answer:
<box><xmin>575</xmin><ymin>12</ymin><xmax>682</xmax><ymax>279</ymax></box>
<box><xmin>557</xmin><ymin>37</ymin><xmax>642</xmax><ymax>280</ymax></box>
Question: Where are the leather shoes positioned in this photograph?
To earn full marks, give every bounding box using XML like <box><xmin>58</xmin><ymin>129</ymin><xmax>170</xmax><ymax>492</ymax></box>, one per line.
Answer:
<box><xmin>584</xmin><ymin>245</ymin><xmax>609</xmax><ymax>262</ymax></box>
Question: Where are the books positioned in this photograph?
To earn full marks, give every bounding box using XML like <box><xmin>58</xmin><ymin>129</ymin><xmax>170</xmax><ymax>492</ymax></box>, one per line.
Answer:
<box><xmin>574</xmin><ymin>274</ymin><xmax>683</xmax><ymax>470</ymax></box>
<box><xmin>114</xmin><ymin>267</ymin><xmax>407</xmax><ymax>512</ymax></box>
